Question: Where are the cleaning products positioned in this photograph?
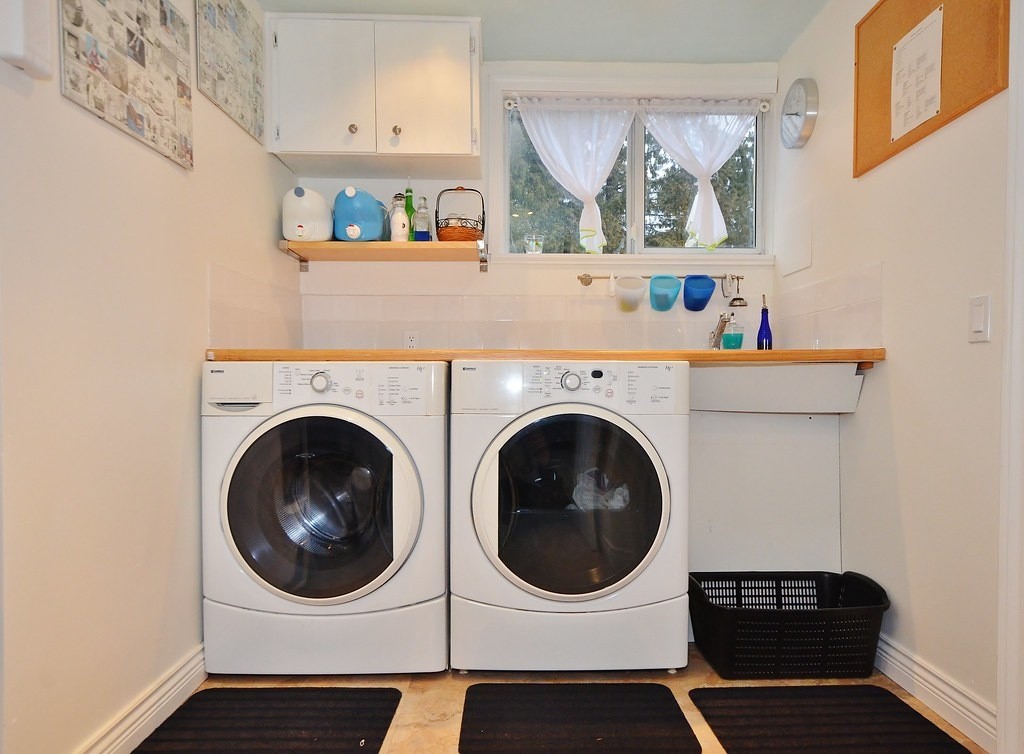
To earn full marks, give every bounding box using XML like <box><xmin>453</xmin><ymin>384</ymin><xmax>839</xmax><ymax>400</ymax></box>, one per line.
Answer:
<box><xmin>389</xmin><ymin>191</ymin><xmax>432</xmax><ymax>241</ymax></box>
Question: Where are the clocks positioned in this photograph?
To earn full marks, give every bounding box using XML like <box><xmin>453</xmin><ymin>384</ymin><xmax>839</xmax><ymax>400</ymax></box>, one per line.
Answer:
<box><xmin>780</xmin><ymin>78</ymin><xmax>820</xmax><ymax>149</ymax></box>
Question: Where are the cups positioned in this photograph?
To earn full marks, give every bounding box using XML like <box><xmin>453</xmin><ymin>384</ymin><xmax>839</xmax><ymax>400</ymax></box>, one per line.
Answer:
<box><xmin>524</xmin><ymin>234</ymin><xmax>544</xmax><ymax>253</ymax></box>
<box><xmin>615</xmin><ymin>276</ymin><xmax>647</xmax><ymax>312</ymax></box>
<box><xmin>684</xmin><ymin>275</ymin><xmax>716</xmax><ymax>311</ymax></box>
<box><xmin>650</xmin><ymin>276</ymin><xmax>682</xmax><ymax>311</ymax></box>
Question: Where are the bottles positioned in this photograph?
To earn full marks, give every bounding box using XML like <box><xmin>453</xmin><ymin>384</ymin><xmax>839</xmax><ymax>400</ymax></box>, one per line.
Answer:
<box><xmin>757</xmin><ymin>295</ymin><xmax>773</xmax><ymax>350</ymax></box>
<box><xmin>334</xmin><ymin>187</ymin><xmax>385</xmax><ymax>241</ymax></box>
<box><xmin>405</xmin><ymin>187</ymin><xmax>416</xmax><ymax>240</ymax></box>
<box><xmin>414</xmin><ymin>196</ymin><xmax>432</xmax><ymax>241</ymax></box>
<box><xmin>391</xmin><ymin>193</ymin><xmax>409</xmax><ymax>241</ymax></box>
<box><xmin>282</xmin><ymin>186</ymin><xmax>333</xmax><ymax>242</ymax></box>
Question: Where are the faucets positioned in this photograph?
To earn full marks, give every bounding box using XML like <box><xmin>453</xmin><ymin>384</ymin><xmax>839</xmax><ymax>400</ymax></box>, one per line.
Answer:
<box><xmin>708</xmin><ymin>312</ymin><xmax>731</xmax><ymax>351</ymax></box>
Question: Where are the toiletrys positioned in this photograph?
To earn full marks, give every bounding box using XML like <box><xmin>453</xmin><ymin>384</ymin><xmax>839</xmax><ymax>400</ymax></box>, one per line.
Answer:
<box><xmin>721</xmin><ymin>312</ymin><xmax>744</xmax><ymax>349</ymax></box>
<box><xmin>756</xmin><ymin>293</ymin><xmax>773</xmax><ymax>349</ymax></box>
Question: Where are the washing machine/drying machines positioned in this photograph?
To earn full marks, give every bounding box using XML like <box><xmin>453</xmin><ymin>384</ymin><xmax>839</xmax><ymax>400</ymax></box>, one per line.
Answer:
<box><xmin>201</xmin><ymin>359</ymin><xmax>450</xmax><ymax>673</ymax></box>
<box><xmin>448</xmin><ymin>360</ymin><xmax>690</xmax><ymax>670</ymax></box>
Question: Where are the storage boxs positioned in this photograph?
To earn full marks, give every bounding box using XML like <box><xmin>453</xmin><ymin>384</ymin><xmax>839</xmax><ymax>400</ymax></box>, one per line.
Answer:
<box><xmin>689</xmin><ymin>569</ymin><xmax>892</xmax><ymax>678</ymax></box>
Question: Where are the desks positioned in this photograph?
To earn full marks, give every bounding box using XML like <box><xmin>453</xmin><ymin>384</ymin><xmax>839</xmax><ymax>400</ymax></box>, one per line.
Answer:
<box><xmin>205</xmin><ymin>347</ymin><xmax>890</xmax><ymax>414</ymax></box>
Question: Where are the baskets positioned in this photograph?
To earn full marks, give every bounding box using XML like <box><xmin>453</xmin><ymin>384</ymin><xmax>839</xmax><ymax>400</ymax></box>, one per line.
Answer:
<box><xmin>435</xmin><ymin>186</ymin><xmax>485</xmax><ymax>242</ymax></box>
<box><xmin>689</xmin><ymin>570</ymin><xmax>891</xmax><ymax>681</ymax></box>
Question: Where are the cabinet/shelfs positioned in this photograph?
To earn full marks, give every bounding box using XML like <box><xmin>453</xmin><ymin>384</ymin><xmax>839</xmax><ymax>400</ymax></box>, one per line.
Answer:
<box><xmin>262</xmin><ymin>10</ymin><xmax>485</xmax><ymax>180</ymax></box>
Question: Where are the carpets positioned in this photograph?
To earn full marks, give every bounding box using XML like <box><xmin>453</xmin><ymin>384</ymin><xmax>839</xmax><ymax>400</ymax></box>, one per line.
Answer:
<box><xmin>689</xmin><ymin>684</ymin><xmax>974</xmax><ymax>754</ymax></box>
<box><xmin>457</xmin><ymin>682</ymin><xmax>704</xmax><ymax>754</ymax></box>
<box><xmin>132</xmin><ymin>687</ymin><xmax>403</xmax><ymax>754</ymax></box>
